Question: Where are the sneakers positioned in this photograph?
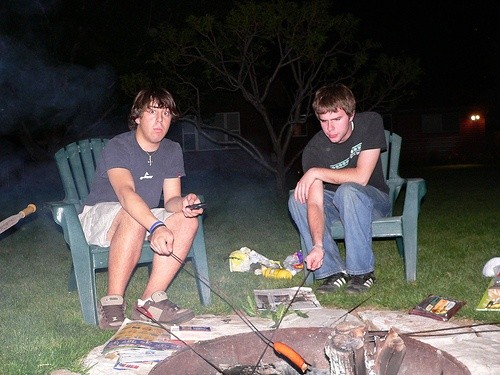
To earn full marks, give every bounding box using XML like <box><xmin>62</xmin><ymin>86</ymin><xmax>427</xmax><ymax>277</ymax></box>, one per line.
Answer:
<box><xmin>131</xmin><ymin>290</ymin><xmax>195</xmax><ymax>326</ymax></box>
<box><xmin>98</xmin><ymin>295</ymin><xmax>126</xmax><ymax>331</ymax></box>
<box><xmin>315</xmin><ymin>272</ymin><xmax>353</xmax><ymax>294</ymax></box>
<box><xmin>344</xmin><ymin>271</ymin><xmax>376</xmax><ymax>295</ymax></box>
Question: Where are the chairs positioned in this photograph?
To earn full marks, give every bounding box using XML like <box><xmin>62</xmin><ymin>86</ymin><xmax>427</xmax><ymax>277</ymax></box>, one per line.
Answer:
<box><xmin>289</xmin><ymin>131</ymin><xmax>427</xmax><ymax>287</ymax></box>
<box><xmin>54</xmin><ymin>138</ymin><xmax>213</xmax><ymax>324</ymax></box>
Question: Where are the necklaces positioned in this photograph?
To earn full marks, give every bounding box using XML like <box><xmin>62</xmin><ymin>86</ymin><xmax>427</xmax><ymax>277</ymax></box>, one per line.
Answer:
<box><xmin>146</xmin><ymin>150</ymin><xmax>155</xmax><ymax>167</ymax></box>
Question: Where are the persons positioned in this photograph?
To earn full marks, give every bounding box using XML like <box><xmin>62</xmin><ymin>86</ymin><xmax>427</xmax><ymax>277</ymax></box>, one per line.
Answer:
<box><xmin>78</xmin><ymin>85</ymin><xmax>203</xmax><ymax>331</ymax></box>
<box><xmin>286</xmin><ymin>83</ymin><xmax>391</xmax><ymax>295</ymax></box>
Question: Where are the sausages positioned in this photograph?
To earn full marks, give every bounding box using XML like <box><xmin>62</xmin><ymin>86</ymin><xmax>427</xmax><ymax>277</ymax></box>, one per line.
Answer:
<box><xmin>274</xmin><ymin>341</ymin><xmax>308</xmax><ymax>373</ymax></box>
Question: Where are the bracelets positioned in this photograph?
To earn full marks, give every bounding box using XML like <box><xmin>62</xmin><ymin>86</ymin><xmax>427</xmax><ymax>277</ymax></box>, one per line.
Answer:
<box><xmin>149</xmin><ymin>221</ymin><xmax>165</xmax><ymax>236</ymax></box>
